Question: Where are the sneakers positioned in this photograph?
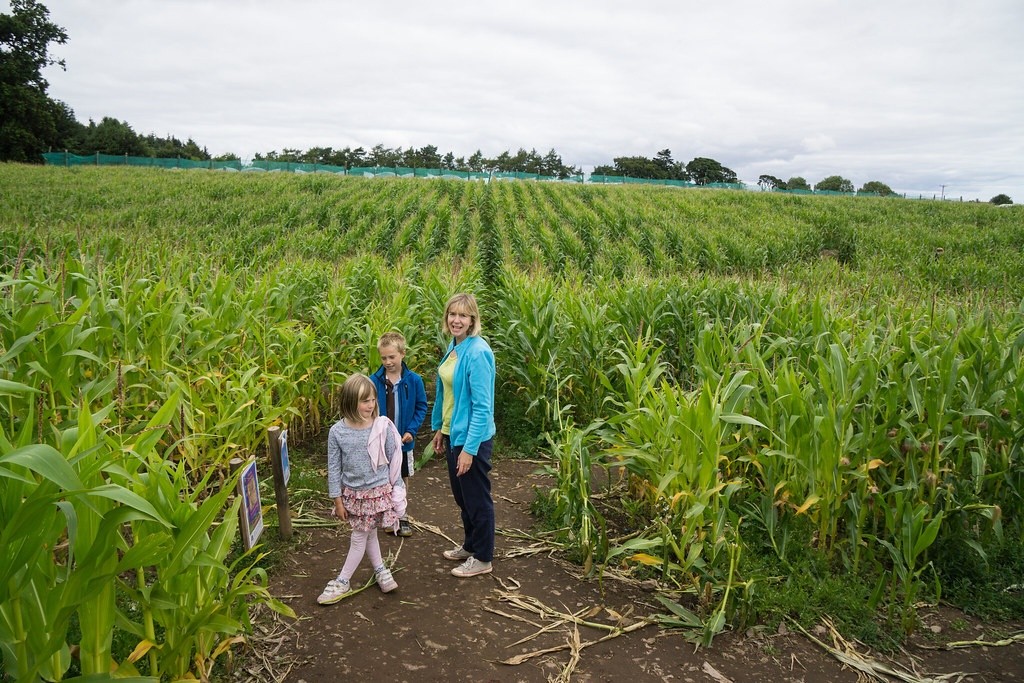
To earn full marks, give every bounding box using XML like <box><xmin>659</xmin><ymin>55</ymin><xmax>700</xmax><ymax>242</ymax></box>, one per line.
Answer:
<box><xmin>398</xmin><ymin>519</ymin><xmax>412</xmax><ymax>537</ymax></box>
<box><xmin>374</xmin><ymin>568</ymin><xmax>398</xmax><ymax>593</ymax></box>
<box><xmin>385</xmin><ymin>525</ymin><xmax>396</xmax><ymax>532</ymax></box>
<box><xmin>316</xmin><ymin>580</ymin><xmax>352</xmax><ymax>604</ymax></box>
<box><xmin>451</xmin><ymin>556</ymin><xmax>492</xmax><ymax>578</ymax></box>
<box><xmin>443</xmin><ymin>544</ymin><xmax>474</xmax><ymax>560</ymax></box>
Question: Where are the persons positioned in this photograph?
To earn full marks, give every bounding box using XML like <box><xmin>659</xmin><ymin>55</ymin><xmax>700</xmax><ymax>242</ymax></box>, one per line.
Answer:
<box><xmin>369</xmin><ymin>333</ymin><xmax>428</xmax><ymax>537</ymax></box>
<box><xmin>431</xmin><ymin>293</ymin><xmax>496</xmax><ymax>577</ymax></box>
<box><xmin>316</xmin><ymin>373</ymin><xmax>407</xmax><ymax>605</ymax></box>
